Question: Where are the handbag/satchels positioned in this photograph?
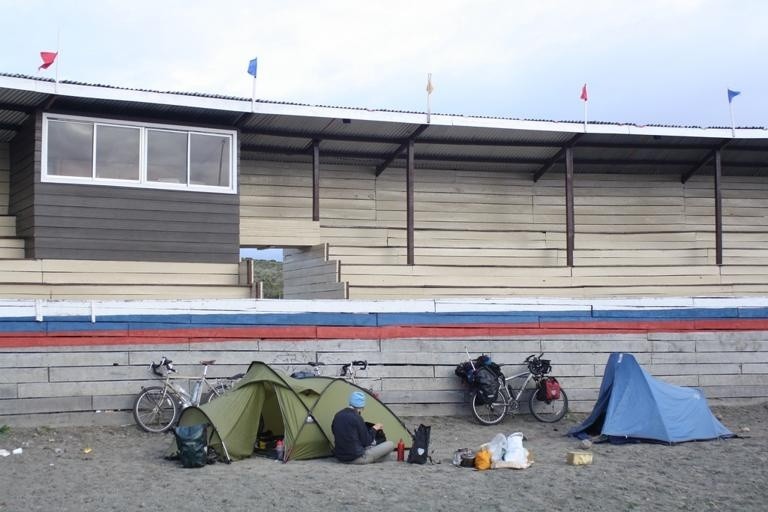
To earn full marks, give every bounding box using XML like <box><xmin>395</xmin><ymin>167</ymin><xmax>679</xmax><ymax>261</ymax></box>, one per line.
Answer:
<box><xmin>364</xmin><ymin>421</ymin><xmax>385</xmax><ymax>450</ymax></box>
<box><xmin>542</xmin><ymin>377</ymin><xmax>560</xmax><ymax>400</ymax></box>
<box><xmin>473</xmin><ymin>367</ymin><xmax>500</xmax><ymax>405</ymax></box>
<box><xmin>406</xmin><ymin>424</ymin><xmax>430</xmax><ymax>465</ymax></box>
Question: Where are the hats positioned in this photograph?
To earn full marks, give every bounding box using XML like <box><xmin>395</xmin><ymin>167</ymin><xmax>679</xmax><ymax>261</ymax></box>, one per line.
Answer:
<box><xmin>348</xmin><ymin>392</ymin><xmax>365</xmax><ymax>408</ymax></box>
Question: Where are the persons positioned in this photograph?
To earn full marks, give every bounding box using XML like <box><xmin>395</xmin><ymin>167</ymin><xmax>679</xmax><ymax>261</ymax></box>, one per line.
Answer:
<box><xmin>330</xmin><ymin>391</ymin><xmax>395</xmax><ymax>466</ymax></box>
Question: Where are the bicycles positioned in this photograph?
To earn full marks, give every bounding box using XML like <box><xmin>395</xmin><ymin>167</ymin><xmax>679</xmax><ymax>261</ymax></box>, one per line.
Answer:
<box><xmin>304</xmin><ymin>357</ymin><xmax>368</xmax><ymax>384</ymax></box>
<box><xmin>131</xmin><ymin>355</ymin><xmax>247</xmax><ymax>435</ymax></box>
<box><xmin>466</xmin><ymin>351</ymin><xmax>570</xmax><ymax>427</ymax></box>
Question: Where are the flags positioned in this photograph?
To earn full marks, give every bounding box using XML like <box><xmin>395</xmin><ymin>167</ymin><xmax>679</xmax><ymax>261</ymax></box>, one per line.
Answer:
<box><xmin>581</xmin><ymin>83</ymin><xmax>588</xmax><ymax>100</ymax></box>
<box><xmin>38</xmin><ymin>50</ymin><xmax>57</xmax><ymax>70</ymax></box>
<box><xmin>246</xmin><ymin>56</ymin><xmax>258</xmax><ymax>81</ymax></box>
<box><xmin>727</xmin><ymin>88</ymin><xmax>741</xmax><ymax>104</ymax></box>
<box><xmin>425</xmin><ymin>75</ymin><xmax>434</xmax><ymax>94</ymax></box>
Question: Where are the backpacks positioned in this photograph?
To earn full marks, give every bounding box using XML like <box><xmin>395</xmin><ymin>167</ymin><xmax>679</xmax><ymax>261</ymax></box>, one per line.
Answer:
<box><xmin>167</xmin><ymin>423</ymin><xmax>214</xmax><ymax>468</ymax></box>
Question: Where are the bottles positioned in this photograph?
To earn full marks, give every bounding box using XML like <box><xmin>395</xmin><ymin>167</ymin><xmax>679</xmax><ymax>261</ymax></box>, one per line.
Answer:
<box><xmin>396</xmin><ymin>437</ymin><xmax>406</xmax><ymax>462</ymax></box>
<box><xmin>170</xmin><ymin>380</ymin><xmax>200</xmax><ymax>404</ymax></box>
<box><xmin>276</xmin><ymin>440</ymin><xmax>284</xmax><ymax>460</ymax></box>
<box><xmin>506</xmin><ymin>383</ymin><xmax>517</xmax><ymax>400</ymax></box>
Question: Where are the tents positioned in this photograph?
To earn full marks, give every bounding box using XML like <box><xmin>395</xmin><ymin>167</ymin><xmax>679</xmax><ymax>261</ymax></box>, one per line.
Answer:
<box><xmin>164</xmin><ymin>359</ymin><xmax>417</xmax><ymax>462</ymax></box>
<box><xmin>567</xmin><ymin>353</ymin><xmax>739</xmax><ymax>445</ymax></box>
